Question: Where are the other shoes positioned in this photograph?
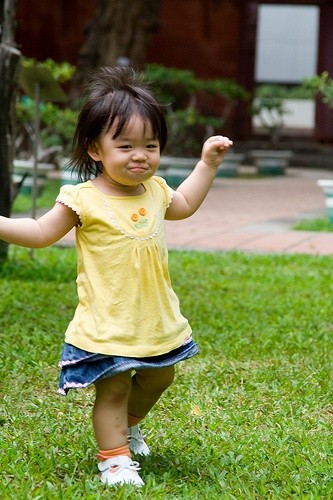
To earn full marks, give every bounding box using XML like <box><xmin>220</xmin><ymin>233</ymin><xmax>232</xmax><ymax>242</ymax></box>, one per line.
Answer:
<box><xmin>126</xmin><ymin>424</ymin><xmax>150</xmax><ymax>457</ymax></box>
<box><xmin>97</xmin><ymin>455</ymin><xmax>146</xmax><ymax>489</ymax></box>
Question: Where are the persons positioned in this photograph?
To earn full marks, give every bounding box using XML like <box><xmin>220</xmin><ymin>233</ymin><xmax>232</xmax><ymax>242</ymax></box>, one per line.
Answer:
<box><xmin>0</xmin><ymin>64</ymin><xmax>233</xmax><ymax>486</ymax></box>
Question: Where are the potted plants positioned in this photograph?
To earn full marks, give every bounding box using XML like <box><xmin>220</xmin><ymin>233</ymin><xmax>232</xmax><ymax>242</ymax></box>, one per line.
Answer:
<box><xmin>8</xmin><ymin>56</ymin><xmax>78</xmax><ymax>197</ymax></box>
<box><xmin>247</xmin><ymin>84</ymin><xmax>296</xmax><ymax>176</ymax></box>
<box><xmin>302</xmin><ymin>70</ymin><xmax>333</xmax><ymax>231</ymax></box>
<box><xmin>60</xmin><ymin>62</ymin><xmax>246</xmax><ymax>192</ymax></box>
